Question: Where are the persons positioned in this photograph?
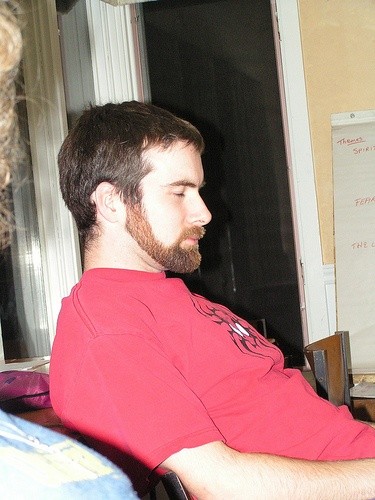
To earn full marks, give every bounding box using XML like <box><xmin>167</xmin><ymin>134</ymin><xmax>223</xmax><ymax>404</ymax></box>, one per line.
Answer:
<box><xmin>50</xmin><ymin>100</ymin><xmax>375</xmax><ymax>500</ymax></box>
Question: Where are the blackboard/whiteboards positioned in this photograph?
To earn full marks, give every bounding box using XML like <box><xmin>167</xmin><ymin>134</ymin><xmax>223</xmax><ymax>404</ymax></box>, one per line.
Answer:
<box><xmin>331</xmin><ymin>108</ymin><xmax>375</xmax><ymax>376</ymax></box>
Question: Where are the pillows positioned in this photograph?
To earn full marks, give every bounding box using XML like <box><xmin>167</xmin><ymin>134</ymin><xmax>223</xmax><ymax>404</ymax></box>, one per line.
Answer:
<box><xmin>0</xmin><ymin>370</ymin><xmax>51</xmax><ymax>407</ymax></box>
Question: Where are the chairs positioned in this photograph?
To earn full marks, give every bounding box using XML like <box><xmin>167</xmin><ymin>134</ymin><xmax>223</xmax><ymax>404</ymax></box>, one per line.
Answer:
<box><xmin>303</xmin><ymin>330</ymin><xmax>351</xmax><ymax>412</ymax></box>
